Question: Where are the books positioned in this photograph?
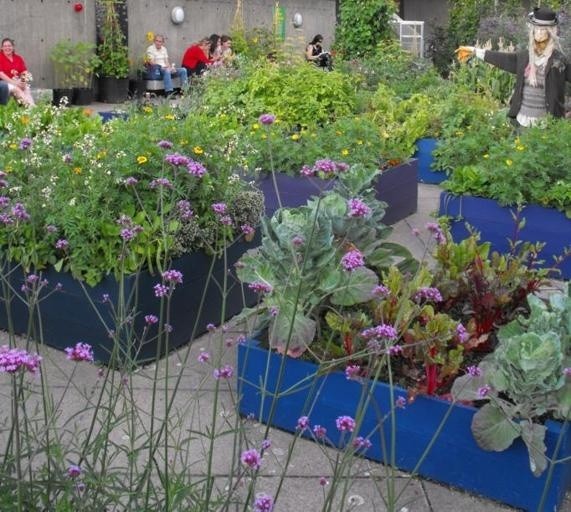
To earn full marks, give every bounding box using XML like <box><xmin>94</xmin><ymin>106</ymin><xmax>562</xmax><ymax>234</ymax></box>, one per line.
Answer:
<box><xmin>318</xmin><ymin>52</ymin><xmax>332</xmax><ymax>56</ymax></box>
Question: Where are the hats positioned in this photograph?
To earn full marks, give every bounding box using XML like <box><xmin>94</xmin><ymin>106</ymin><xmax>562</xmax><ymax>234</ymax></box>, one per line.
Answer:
<box><xmin>525</xmin><ymin>6</ymin><xmax>559</xmax><ymax>27</ymax></box>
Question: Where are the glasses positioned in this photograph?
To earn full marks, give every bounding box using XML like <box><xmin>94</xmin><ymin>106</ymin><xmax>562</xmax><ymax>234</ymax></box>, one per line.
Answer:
<box><xmin>319</xmin><ymin>41</ymin><xmax>322</xmax><ymax>43</ymax></box>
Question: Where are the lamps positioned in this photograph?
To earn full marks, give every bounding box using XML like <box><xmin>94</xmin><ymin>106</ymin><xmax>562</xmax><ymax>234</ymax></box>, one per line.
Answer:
<box><xmin>172</xmin><ymin>7</ymin><xmax>185</xmax><ymax>25</ymax></box>
<box><xmin>293</xmin><ymin>11</ymin><xmax>303</xmax><ymax>27</ymax></box>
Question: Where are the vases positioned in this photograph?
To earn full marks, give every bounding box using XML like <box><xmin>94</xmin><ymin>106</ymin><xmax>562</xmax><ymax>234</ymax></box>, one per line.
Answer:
<box><xmin>254</xmin><ymin>171</ymin><xmax>420</xmax><ymax>230</ymax></box>
<box><xmin>417</xmin><ymin>139</ymin><xmax>568</xmax><ymax>281</ymax></box>
<box><xmin>0</xmin><ymin>222</ymin><xmax>266</xmax><ymax>374</ymax></box>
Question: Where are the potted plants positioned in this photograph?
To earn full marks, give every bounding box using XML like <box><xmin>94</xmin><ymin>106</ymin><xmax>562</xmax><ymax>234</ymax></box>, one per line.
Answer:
<box><xmin>51</xmin><ymin>2</ymin><xmax>130</xmax><ymax>107</ymax></box>
<box><xmin>234</xmin><ymin>163</ymin><xmax>569</xmax><ymax>512</ymax></box>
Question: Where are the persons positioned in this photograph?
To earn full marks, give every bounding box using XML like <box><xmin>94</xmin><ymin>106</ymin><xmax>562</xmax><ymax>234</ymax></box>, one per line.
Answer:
<box><xmin>209</xmin><ymin>33</ymin><xmax>226</xmax><ymax>68</ymax></box>
<box><xmin>0</xmin><ymin>38</ymin><xmax>34</xmax><ymax>108</ymax></box>
<box><xmin>181</xmin><ymin>37</ymin><xmax>221</xmax><ymax>75</ymax></box>
<box><xmin>221</xmin><ymin>35</ymin><xmax>234</xmax><ymax>62</ymax></box>
<box><xmin>453</xmin><ymin>9</ymin><xmax>571</xmax><ymax>138</ymax></box>
<box><xmin>0</xmin><ymin>80</ymin><xmax>29</xmax><ymax>108</ymax></box>
<box><xmin>306</xmin><ymin>34</ymin><xmax>332</xmax><ymax>71</ymax></box>
<box><xmin>145</xmin><ymin>34</ymin><xmax>189</xmax><ymax>98</ymax></box>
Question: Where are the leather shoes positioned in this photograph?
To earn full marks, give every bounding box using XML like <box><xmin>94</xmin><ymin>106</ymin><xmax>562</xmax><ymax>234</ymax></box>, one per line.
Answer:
<box><xmin>165</xmin><ymin>92</ymin><xmax>176</xmax><ymax>100</ymax></box>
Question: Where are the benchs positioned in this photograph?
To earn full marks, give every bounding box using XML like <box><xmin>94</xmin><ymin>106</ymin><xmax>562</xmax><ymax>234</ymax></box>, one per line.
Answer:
<box><xmin>147</xmin><ymin>76</ymin><xmax>197</xmax><ymax>98</ymax></box>
<box><xmin>0</xmin><ymin>80</ymin><xmax>50</xmax><ymax>105</ymax></box>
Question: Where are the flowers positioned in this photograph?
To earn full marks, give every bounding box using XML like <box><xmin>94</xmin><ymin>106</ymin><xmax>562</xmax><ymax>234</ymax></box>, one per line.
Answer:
<box><xmin>0</xmin><ymin>100</ymin><xmax>266</xmax><ymax>293</ymax></box>
<box><xmin>233</xmin><ymin>104</ymin><xmax>426</xmax><ymax>177</ymax></box>
<box><xmin>421</xmin><ymin>88</ymin><xmax>570</xmax><ymax>210</ymax></box>
